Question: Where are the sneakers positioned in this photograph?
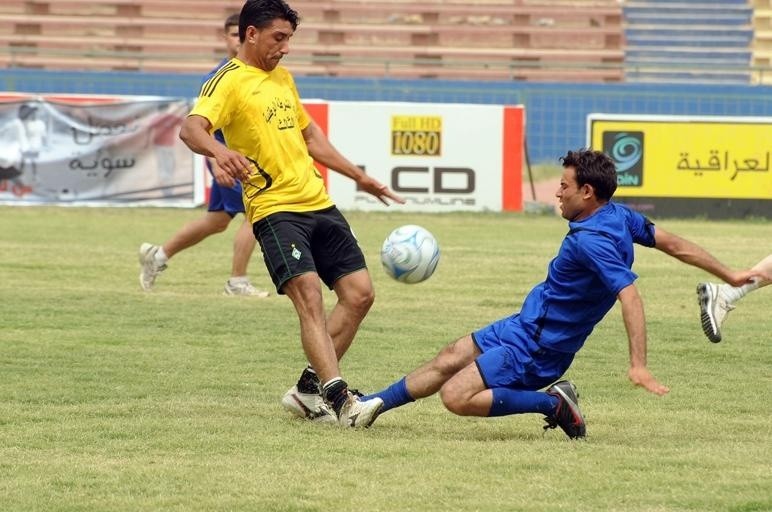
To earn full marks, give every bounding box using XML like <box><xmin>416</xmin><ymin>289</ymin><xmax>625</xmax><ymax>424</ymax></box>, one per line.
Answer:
<box><xmin>222</xmin><ymin>275</ymin><xmax>272</xmax><ymax>300</ymax></box>
<box><xmin>543</xmin><ymin>378</ymin><xmax>587</xmax><ymax>440</ymax></box>
<box><xmin>138</xmin><ymin>242</ymin><xmax>168</xmax><ymax>294</ymax></box>
<box><xmin>280</xmin><ymin>383</ymin><xmax>385</xmax><ymax>432</ymax></box>
<box><xmin>696</xmin><ymin>280</ymin><xmax>738</xmax><ymax>344</ymax></box>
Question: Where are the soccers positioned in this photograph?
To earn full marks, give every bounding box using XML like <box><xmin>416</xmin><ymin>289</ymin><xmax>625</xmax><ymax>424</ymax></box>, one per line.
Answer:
<box><xmin>382</xmin><ymin>224</ymin><xmax>439</xmax><ymax>282</ymax></box>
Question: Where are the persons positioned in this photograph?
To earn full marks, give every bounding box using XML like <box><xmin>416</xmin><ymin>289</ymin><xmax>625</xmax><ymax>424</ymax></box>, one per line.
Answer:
<box><xmin>179</xmin><ymin>0</ymin><xmax>409</xmax><ymax>425</ymax></box>
<box><xmin>349</xmin><ymin>148</ymin><xmax>771</xmax><ymax>442</ymax></box>
<box><xmin>140</xmin><ymin>13</ymin><xmax>274</xmax><ymax>299</ymax></box>
<box><xmin>695</xmin><ymin>251</ymin><xmax>770</xmax><ymax>344</ymax></box>
<box><xmin>0</xmin><ymin>104</ymin><xmax>40</xmax><ymax>195</ymax></box>
